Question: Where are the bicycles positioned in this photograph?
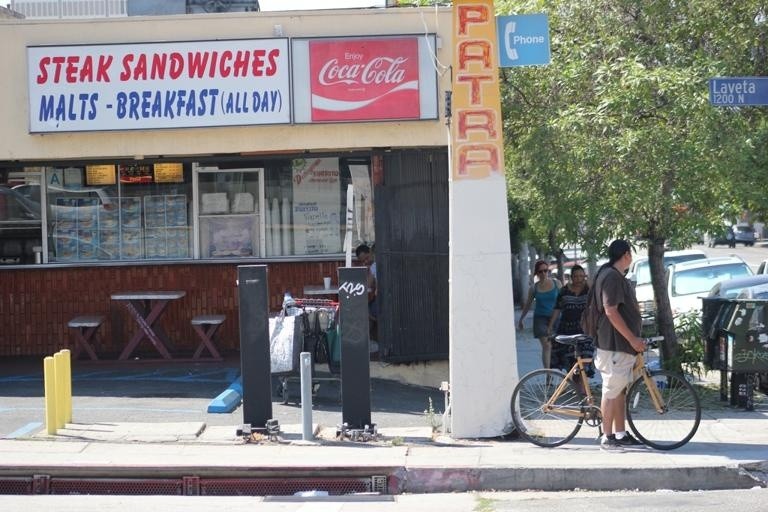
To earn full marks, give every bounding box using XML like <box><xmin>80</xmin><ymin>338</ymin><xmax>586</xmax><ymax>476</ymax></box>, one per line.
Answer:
<box><xmin>510</xmin><ymin>334</ymin><xmax>701</xmax><ymax>450</ymax></box>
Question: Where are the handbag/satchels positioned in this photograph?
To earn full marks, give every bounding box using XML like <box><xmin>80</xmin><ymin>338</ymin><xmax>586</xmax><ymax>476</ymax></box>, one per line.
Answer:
<box><xmin>270</xmin><ymin>309</ymin><xmax>341</xmax><ymax>374</ymax></box>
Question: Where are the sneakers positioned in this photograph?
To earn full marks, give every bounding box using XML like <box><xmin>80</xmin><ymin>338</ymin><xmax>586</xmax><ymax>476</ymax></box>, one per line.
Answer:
<box><xmin>601</xmin><ymin>434</ymin><xmax>624</xmax><ymax>453</ymax></box>
<box><xmin>615</xmin><ymin>431</ymin><xmax>644</xmax><ymax>448</ymax></box>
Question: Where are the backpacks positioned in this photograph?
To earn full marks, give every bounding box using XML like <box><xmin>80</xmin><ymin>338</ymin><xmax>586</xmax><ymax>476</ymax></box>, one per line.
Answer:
<box><xmin>580</xmin><ymin>286</ymin><xmax>601</xmax><ymax>337</ymax></box>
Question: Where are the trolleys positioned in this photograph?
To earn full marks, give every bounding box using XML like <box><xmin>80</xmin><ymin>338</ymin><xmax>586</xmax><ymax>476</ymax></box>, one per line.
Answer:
<box><xmin>279</xmin><ymin>298</ymin><xmax>342</xmax><ymax>404</ymax></box>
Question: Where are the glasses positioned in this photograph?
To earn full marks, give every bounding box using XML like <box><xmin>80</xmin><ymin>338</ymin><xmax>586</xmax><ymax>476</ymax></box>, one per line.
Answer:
<box><xmin>538</xmin><ymin>270</ymin><xmax>548</xmax><ymax>273</ymax></box>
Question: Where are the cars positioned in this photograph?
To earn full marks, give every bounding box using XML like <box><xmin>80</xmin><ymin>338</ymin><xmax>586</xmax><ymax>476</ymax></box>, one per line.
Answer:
<box><xmin>518</xmin><ymin>250</ymin><xmax>768</xmax><ymax>338</ymax></box>
<box><xmin>694</xmin><ymin>221</ymin><xmax>754</xmax><ymax>248</ymax></box>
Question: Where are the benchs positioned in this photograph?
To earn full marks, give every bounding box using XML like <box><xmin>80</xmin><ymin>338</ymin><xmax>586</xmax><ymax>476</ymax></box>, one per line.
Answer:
<box><xmin>191</xmin><ymin>314</ymin><xmax>225</xmax><ymax>358</ymax></box>
<box><xmin>67</xmin><ymin>314</ymin><xmax>106</xmax><ymax>361</ymax></box>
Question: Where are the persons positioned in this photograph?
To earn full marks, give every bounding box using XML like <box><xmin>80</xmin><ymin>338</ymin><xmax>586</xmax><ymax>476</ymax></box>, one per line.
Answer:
<box><xmin>356</xmin><ymin>246</ymin><xmax>371</xmax><ymax>266</ymax></box>
<box><xmin>368</xmin><ymin>244</ymin><xmax>377</xmax><ymax>321</ymax></box>
<box><xmin>547</xmin><ymin>265</ymin><xmax>595</xmax><ymax>394</ymax></box>
<box><xmin>590</xmin><ymin>239</ymin><xmax>647</xmax><ymax>451</ymax></box>
<box><xmin>519</xmin><ymin>261</ymin><xmax>563</xmax><ymax>388</ymax></box>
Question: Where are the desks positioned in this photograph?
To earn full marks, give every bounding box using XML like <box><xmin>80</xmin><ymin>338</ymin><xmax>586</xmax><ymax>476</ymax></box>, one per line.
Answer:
<box><xmin>303</xmin><ymin>284</ymin><xmax>339</xmax><ymax>303</ymax></box>
<box><xmin>110</xmin><ymin>291</ymin><xmax>187</xmax><ymax>360</ymax></box>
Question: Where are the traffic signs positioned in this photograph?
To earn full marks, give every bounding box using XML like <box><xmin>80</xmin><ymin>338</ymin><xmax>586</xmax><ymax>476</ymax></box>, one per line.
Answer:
<box><xmin>709</xmin><ymin>76</ymin><xmax>768</xmax><ymax>105</ymax></box>
